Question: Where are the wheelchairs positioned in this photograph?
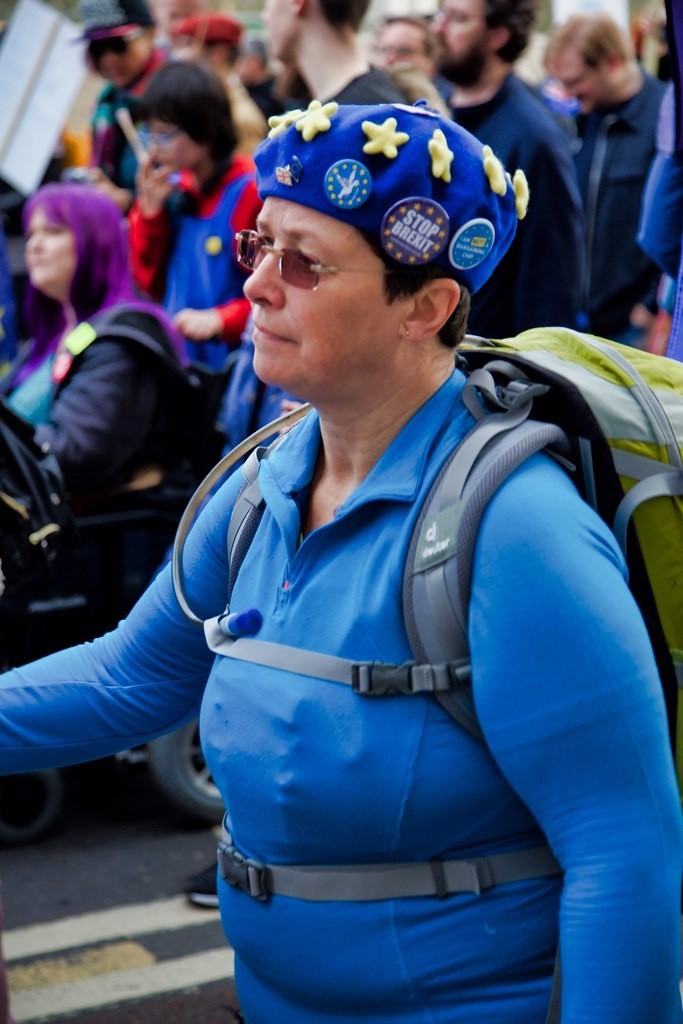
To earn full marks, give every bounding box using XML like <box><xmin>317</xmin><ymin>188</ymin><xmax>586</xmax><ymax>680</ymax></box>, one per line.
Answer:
<box><xmin>0</xmin><ymin>363</ymin><xmax>225</xmax><ymax>849</ymax></box>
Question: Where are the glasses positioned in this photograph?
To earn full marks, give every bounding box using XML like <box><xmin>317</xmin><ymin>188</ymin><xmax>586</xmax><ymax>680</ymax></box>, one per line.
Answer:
<box><xmin>137</xmin><ymin>123</ymin><xmax>183</xmax><ymax>158</ymax></box>
<box><xmin>235</xmin><ymin>229</ymin><xmax>412</xmax><ymax>290</ymax></box>
<box><xmin>88</xmin><ymin>29</ymin><xmax>145</xmax><ymax>58</ymax></box>
<box><xmin>373</xmin><ymin>43</ymin><xmax>423</xmax><ymax>61</ymax></box>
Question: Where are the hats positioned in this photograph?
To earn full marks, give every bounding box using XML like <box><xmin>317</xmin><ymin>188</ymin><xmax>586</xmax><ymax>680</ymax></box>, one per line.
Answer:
<box><xmin>67</xmin><ymin>0</ymin><xmax>156</xmax><ymax>43</ymax></box>
<box><xmin>174</xmin><ymin>15</ymin><xmax>242</xmax><ymax>49</ymax></box>
<box><xmin>253</xmin><ymin>99</ymin><xmax>531</xmax><ymax>295</ymax></box>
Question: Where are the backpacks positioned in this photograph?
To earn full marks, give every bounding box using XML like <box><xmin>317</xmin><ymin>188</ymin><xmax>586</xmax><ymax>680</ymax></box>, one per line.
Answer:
<box><xmin>185</xmin><ymin>320</ymin><xmax>683</xmax><ymax>902</ymax></box>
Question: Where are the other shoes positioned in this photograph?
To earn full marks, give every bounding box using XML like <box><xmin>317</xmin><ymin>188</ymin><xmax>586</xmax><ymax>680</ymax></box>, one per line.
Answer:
<box><xmin>184</xmin><ymin>864</ymin><xmax>220</xmax><ymax>909</ymax></box>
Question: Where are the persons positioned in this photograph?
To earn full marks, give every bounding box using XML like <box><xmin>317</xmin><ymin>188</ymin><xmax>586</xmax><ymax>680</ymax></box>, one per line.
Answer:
<box><xmin>0</xmin><ymin>0</ymin><xmax>683</xmax><ymax>906</ymax></box>
<box><xmin>0</xmin><ymin>100</ymin><xmax>682</xmax><ymax>1024</ymax></box>
